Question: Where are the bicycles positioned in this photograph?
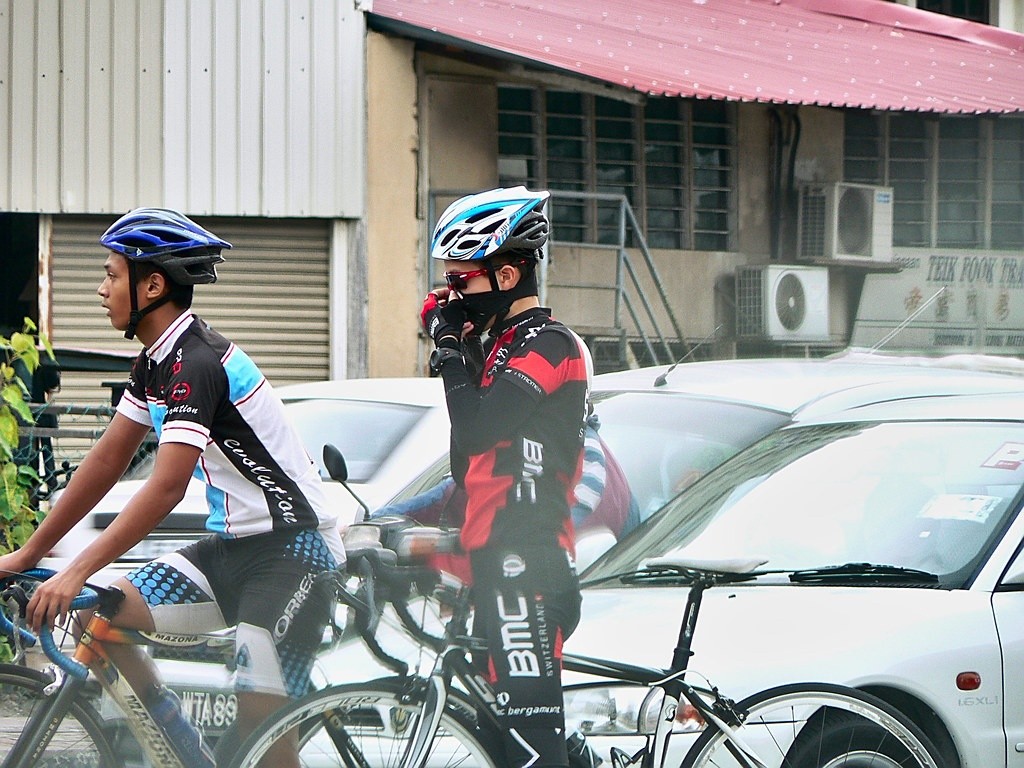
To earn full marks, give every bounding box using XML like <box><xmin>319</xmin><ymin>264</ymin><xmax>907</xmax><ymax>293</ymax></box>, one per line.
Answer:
<box><xmin>229</xmin><ymin>444</ymin><xmax>948</xmax><ymax>768</ymax></box>
<box><xmin>0</xmin><ymin>567</ymin><xmax>477</xmax><ymax>768</ymax></box>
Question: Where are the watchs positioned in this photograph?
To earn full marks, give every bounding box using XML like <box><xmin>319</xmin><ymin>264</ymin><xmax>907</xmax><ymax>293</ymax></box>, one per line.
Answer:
<box><xmin>430</xmin><ymin>348</ymin><xmax>463</xmax><ymax>372</ymax></box>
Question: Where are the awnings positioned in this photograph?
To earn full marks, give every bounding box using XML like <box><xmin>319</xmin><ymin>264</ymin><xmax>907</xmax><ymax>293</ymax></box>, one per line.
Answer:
<box><xmin>368</xmin><ymin>0</ymin><xmax>1024</xmax><ymax>117</ymax></box>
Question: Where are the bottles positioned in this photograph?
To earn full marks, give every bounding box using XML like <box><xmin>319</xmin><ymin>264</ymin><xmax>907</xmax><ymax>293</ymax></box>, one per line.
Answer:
<box><xmin>146</xmin><ymin>684</ymin><xmax>217</xmax><ymax>768</ymax></box>
<box><xmin>565</xmin><ymin>728</ymin><xmax>603</xmax><ymax>768</ymax></box>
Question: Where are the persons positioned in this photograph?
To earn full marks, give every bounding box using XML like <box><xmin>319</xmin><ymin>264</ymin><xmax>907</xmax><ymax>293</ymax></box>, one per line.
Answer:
<box><xmin>0</xmin><ymin>207</ymin><xmax>346</xmax><ymax>768</ymax></box>
<box><xmin>338</xmin><ymin>187</ymin><xmax>592</xmax><ymax>768</ymax></box>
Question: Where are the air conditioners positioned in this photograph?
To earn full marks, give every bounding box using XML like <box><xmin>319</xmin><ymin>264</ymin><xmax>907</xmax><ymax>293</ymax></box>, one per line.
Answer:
<box><xmin>732</xmin><ymin>262</ymin><xmax>830</xmax><ymax>343</ymax></box>
<box><xmin>796</xmin><ymin>181</ymin><xmax>894</xmax><ymax>263</ymax></box>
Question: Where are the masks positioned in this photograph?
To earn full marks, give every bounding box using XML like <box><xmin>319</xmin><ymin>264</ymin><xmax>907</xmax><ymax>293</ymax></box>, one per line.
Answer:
<box><xmin>457</xmin><ymin>290</ymin><xmax>515</xmax><ymax>337</ymax></box>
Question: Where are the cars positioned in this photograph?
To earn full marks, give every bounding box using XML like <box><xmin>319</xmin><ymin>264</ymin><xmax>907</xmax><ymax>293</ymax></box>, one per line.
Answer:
<box><xmin>48</xmin><ymin>349</ymin><xmax>1023</xmax><ymax>766</ymax></box>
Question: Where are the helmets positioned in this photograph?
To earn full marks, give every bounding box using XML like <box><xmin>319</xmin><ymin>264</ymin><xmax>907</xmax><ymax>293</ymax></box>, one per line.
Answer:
<box><xmin>429</xmin><ymin>185</ymin><xmax>551</xmax><ymax>262</ymax></box>
<box><xmin>99</xmin><ymin>205</ymin><xmax>233</xmax><ymax>286</ymax></box>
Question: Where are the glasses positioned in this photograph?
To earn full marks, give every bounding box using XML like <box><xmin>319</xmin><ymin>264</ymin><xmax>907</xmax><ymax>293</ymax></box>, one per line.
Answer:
<box><xmin>442</xmin><ymin>258</ymin><xmax>527</xmax><ymax>292</ymax></box>
